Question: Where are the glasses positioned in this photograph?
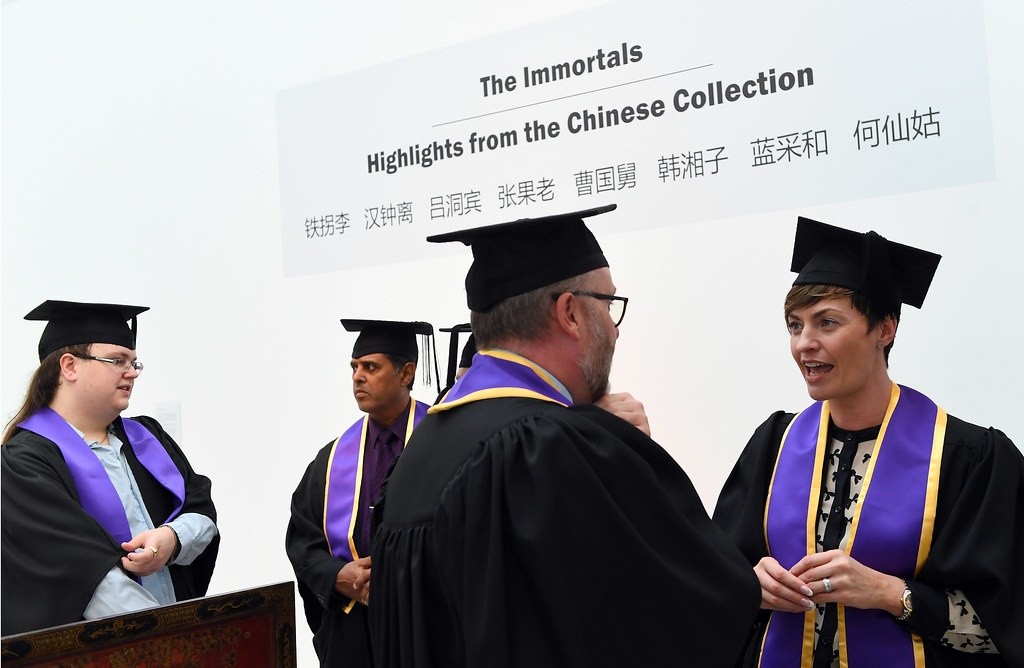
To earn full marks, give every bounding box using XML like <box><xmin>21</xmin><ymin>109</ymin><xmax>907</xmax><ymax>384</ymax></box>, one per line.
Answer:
<box><xmin>549</xmin><ymin>291</ymin><xmax>629</xmax><ymax>328</ymax></box>
<box><xmin>65</xmin><ymin>352</ymin><xmax>143</xmax><ymax>375</ymax></box>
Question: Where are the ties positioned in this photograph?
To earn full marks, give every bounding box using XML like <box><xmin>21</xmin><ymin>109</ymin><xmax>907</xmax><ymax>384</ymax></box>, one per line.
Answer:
<box><xmin>374</xmin><ymin>428</ymin><xmax>395</xmax><ymax>510</ymax></box>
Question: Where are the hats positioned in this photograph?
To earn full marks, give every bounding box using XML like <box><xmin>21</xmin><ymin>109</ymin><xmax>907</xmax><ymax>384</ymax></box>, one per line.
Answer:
<box><xmin>426</xmin><ymin>204</ymin><xmax>617</xmax><ymax>313</ymax></box>
<box><xmin>790</xmin><ymin>216</ymin><xmax>942</xmax><ymax>310</ymax></box>
<box><xmin>340</xmin><ymin>319</ymin><xmax>441</xmax><ymax>395</ymax></box>
<box><xmin>439</xmin><ymin>323</ymin><xmax>477</xmax><ymax>389</ymax></box>
<box><xmin>23</xmin><ymin>300</ymin><xmax>150</xmax><ymax>364</ymax></box>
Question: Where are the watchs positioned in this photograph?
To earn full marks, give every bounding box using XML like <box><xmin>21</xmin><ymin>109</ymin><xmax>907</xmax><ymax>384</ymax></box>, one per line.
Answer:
<box><xmin>896</xmin><ymin>577</ymin><xmax>914</xmax><ymax>623</ymax></box>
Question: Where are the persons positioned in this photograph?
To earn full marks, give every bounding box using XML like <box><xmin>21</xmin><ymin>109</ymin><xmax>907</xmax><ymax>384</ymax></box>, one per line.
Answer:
<box><xmin>0</xmin><ymin>299</ymin><xmax>222</xmax><ymax>639</ymax></box>
<box><xmin>710</xmin><ymin>216</ymin><xmax>1024</xmax><ymax>668</ymax></box>
<box><xmin>284</xmin><ymin>318</ymin><xmax>437</xmax><ymax>668</ymax></box>
<box><xmin>436</xmin><ymin>323</ymin><xmax>476</xmax><ymax>384</ymax></box>
<box><xmin>367</xmin><ymin>200</ymin><xmax>761</xmax><ymax>668</ymax></box>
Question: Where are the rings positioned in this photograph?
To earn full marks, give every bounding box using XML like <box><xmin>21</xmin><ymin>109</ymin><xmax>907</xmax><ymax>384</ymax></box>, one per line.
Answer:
<box><xmin>822</xmin><ymin>577</ymin><xmax>832</xmax><ymax>593</ymax></box>
<box><xmin>148</xmin><ymin>545</ymin><xmax>159</xmax><ymax>559</ymax></box>
